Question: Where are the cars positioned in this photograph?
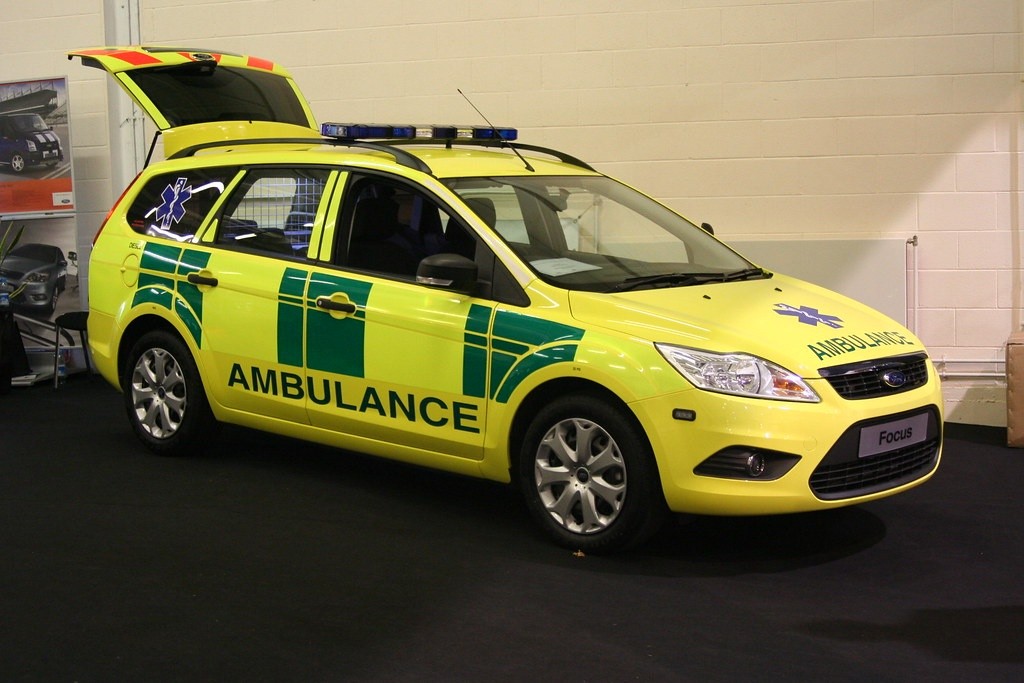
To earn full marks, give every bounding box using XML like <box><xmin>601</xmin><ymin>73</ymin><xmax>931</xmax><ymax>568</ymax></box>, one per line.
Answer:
<box><xmin>1</xmin><ymin>243</ymin><xmax>68</xmax><ymax>313</ymax></box>
<box><xmin>67</xmin><ymin>46</ymin><xmax>943</xmax><ymax>551</ymax></box>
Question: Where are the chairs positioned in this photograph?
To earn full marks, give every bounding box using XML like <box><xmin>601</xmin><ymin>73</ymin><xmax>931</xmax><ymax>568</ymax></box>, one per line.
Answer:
<box><xmin>443</xmin><ymin>197</ymin><xmax>507</xmax><ymax>262</ymax></box>
<box><xmin>335</xmin><ymin>176</ymin><xmax>418</xmax><ymax>278</ymax></box>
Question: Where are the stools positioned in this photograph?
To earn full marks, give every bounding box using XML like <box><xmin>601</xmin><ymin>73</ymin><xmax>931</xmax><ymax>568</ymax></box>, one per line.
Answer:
<box><xmin>53</xmin><ymin>312</ymin><xmax>89</xmax><ymax>391</ymax></box>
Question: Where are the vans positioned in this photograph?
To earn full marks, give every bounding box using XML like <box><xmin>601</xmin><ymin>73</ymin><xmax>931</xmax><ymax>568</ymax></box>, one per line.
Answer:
<box><xmin>0</xmin><ymin>113</ymin><xmax>63</xmax><ymax>172</ymax></box>
